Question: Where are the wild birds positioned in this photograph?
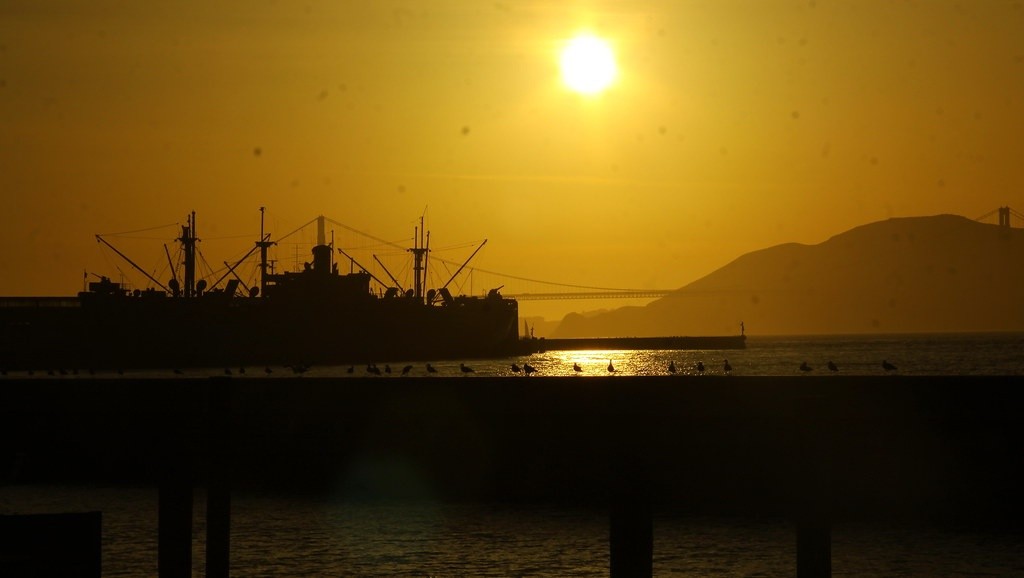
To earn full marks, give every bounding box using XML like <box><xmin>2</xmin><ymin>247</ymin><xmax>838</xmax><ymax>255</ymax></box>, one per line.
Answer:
<box><xmin>459</xmin><ymin>363</ymin><xmax>476</xmax><ymax>375</ymax></box>
<box><xmin>511</xmin><ymin>364</ymin><xmax>521</xmax><ymax>373</ymax></box>
<box><xmin>800</xmin><ymin>362</ymin><xmax>813</xmax><ymax>372</ymax></box>
<box><xmin>698</xmin><ymin>361</ymin><xmax>705</xmax><ymax>373</ymax></box>
<box><xmin>264</xmin><ymin>366</ymin><xmax>274</xmax><ymax>375</ymax></box>
<box><xmin>239</xmin><ymin>367</ymin><xmax>247</xmax><ymax>375</ymax></box>
<box><xmin>607</xmin><ymin>359</ymin><xmax>614</xmax><ymax>372</ymax></box>
<box><xmin>573</xmin><ymin>363</ymin><xmax>583</xmax><ymax>374</ymax></box>
<box><xmin>523</xmin><ymin>363</ymin><xmax>539</xmax><ymax>375</ymax></box>
<box><xmin>347</xmin><ymin>362</ymin><xmax>437</xmax><ymax>375</ymax></box>
<box><xmin>225</xmin><ymin>365</ymin><xmax>232</xmax><ymax>375</ymax></box>
<box><xmin>668</xmin><ymin>361</ymin><xmax>677</xmax><ymax>374</ymax></box>
<box><xmin>881</xmin><ymin>360</ymin><xmax>899</xmax><ymax>371</ymax></box>
<box><xmin>0</xmin><ymin>367</ymin><xmax>125</xmax><ymax>378</ymax></box>
<box><xmin>827</xmin><ymin>361</ymin><xmax>839</xmax><ymax>373</ymax></box>
<box><xmin>722</xmin><ymin>359</ymin><xmax>732</xmax><ymax>373</ymax></box>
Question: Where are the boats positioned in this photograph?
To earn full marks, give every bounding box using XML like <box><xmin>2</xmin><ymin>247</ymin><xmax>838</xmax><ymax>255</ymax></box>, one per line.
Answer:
<box><xmin>50</xmin><ymin>206</ymin><xmax>548</xmax><ymax>371</ymax></box>
